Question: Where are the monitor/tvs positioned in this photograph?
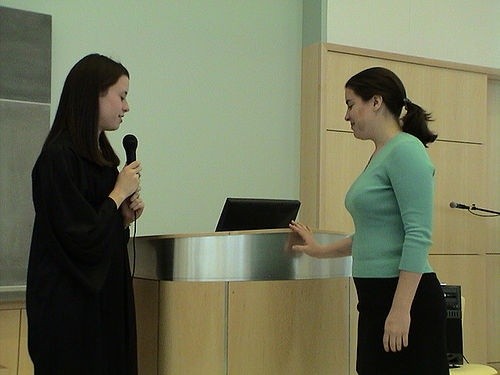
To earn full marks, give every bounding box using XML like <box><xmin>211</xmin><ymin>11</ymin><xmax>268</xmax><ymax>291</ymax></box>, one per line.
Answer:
<box><xmin>215</xmin><ymin>198</ymin><xmax>301</xmax><ymax>233</ymax></box>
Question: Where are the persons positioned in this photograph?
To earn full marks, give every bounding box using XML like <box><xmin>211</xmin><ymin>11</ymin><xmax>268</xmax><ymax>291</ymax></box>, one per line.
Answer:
<box><xmin>290</xmin><ymin>67</ymin><xmax>448</xmax><ymax>375</ymax></box>
<box><xmin>26</xmin><ymin>54</ymin><xmax>144</xmax><ymax>375</ymax></box>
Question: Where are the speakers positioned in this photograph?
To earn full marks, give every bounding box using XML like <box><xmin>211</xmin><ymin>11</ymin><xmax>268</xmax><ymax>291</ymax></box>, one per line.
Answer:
<box><xmin>442</xmin><ymin>284</ymin><xmax>463</xmax><ymax>366</ymax></box>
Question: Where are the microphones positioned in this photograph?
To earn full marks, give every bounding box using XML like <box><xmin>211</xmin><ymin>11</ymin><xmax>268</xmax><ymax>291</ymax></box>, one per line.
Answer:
<box><xmin>450</xmin><ymin>202</ymin><xmax>476</xmax><ymax>210</ymax></box>
<box><xmin>123</xmin><ymin>134</ymin><xmax>138</xmax><ymax>166</ymax></box>
